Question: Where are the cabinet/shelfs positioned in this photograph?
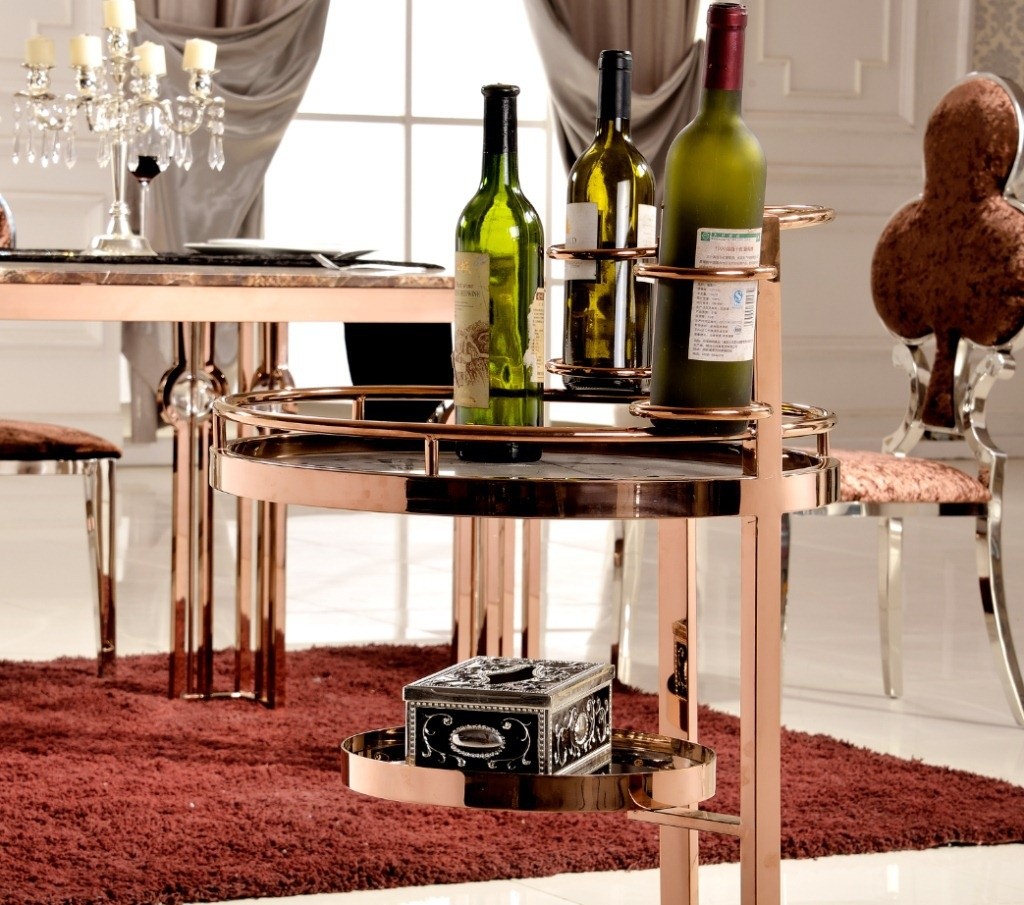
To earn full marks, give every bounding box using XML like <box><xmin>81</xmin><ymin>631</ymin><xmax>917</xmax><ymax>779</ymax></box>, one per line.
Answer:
<box><xmin>210</xmin><ymin>204</ymin><xmax>843</xmax><ymax>905</ymax></box>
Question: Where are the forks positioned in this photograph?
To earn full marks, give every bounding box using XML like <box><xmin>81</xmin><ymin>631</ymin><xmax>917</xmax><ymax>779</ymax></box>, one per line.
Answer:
<box><xmin>309</xmin><ymin>253</ymin><xmax>444</xmax><ymax>271</ymax></box>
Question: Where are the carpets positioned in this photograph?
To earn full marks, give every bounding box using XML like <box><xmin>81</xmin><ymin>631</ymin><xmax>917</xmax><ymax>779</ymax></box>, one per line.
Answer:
<box><xmin>0</xmin><ymin>641</ymin><xmax>1024</xmax><ymax>905</ymax></box>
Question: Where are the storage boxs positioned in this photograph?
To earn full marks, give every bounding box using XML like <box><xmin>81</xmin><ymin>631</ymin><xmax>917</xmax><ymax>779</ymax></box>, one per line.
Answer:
<box><xmin>403</xmin><ymin>654</ymin><xmax>616</xmax><ymax>776</ymax></box>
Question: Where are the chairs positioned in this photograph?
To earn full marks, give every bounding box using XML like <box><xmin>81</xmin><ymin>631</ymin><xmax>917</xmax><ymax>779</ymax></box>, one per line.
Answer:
<box><xmin>0</xmin><ymin>417</ymin><xmax>125</xmax><ymax>680</ymax></box>
<box><xmin>781</xmin><ymin>68</ymin><xmax>1024</xmax><ymax>731</ymax></box>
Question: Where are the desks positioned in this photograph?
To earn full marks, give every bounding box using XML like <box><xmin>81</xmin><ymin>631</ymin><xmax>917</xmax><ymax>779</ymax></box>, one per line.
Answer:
<box><xmin>0</xmin><ymin>263</ymin><xmax>555</xmax><ymax>707</ymax></box>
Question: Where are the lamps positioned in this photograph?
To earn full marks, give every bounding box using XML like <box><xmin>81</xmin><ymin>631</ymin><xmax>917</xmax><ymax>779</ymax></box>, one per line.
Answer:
<box><xmin>124</xmin><ymin>155</ymin><xmax>173</xmax><ymax>237</ymax></box>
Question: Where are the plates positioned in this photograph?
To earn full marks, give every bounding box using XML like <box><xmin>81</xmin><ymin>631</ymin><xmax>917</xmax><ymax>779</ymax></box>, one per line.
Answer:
<box><xmin>184</xmin><ymin>242</ymin><xmax>374</xmax><ymax>260</ymax></box>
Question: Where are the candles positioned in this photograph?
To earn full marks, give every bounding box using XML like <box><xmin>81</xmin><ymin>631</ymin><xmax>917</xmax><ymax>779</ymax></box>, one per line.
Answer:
<box><xmin>104</xmin><ymin>0</ymin><xmax>137</xmax><ymax>33</ymax></box>
<box><xmin>24</xmin><ymin>32</ymin><xmax>55</xmax><ymax>66</ymax></box>
<box><xmin>69</xmin><ymin>33</ymin><xmax>103</xmax><ymax>68</ymax></box>
<box><xmin>134</xmin><ymin>39</ymin><xmax>168</xmax><ymax>80</ymax></box>
<box><xmin>182</xmin><ymin>37</ymin><xmax>218</xmax><ymax>74</ymax></box>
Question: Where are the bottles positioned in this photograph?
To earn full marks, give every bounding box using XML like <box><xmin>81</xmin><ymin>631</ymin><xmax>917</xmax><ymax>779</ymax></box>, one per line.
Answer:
<box><xmin>562</xmin><ymin>51</ymin><xmax>657</xmax><ymax>395</ymax></box>
<box><xmin>452</xmin><ymin>83</ymin><xmax>546</xmax><ymax>462</ymax></box>
<box><xmin>646</xmin><ymin>0</ymin><xmax>765</xmax><ymax>436</ymax></box>
